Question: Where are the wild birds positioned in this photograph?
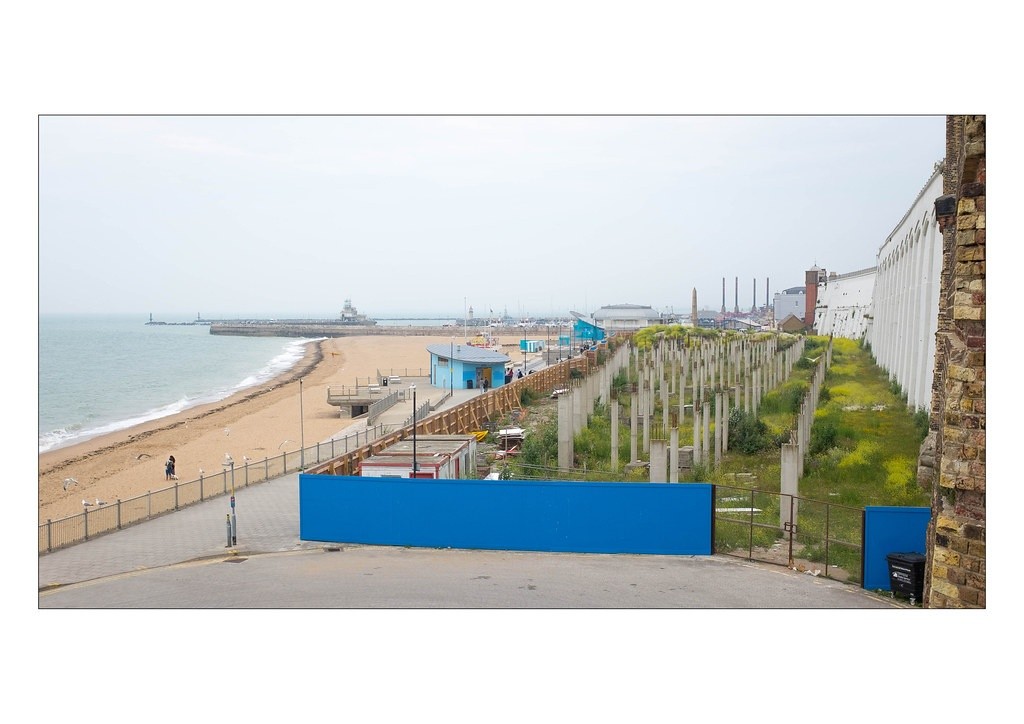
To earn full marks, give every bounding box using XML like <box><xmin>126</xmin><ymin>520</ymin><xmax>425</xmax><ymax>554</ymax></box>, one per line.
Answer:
<box><xmin>278</xmin><ymin>439</ymin><xmax>296</xmax><ymax>448</ymax></box>
<box><xmin>95</xmin><ymin>499</ymin><xmax>108</xmax><ymax>507</ymax></box>
<box><xmin>82</xmin><ymin>500</ymin><xmax>94</xmax><ymax>508</ymax></box>
<box><xmin>224</xmin><ymin>428</ymin><xmax>231</xmax><ymax>435</ymax></box>
<box><xmin>199</xmin><ymin>467</ymin><xmax>205</xmax><ymax>475</ymax></box>
<box><xmin>225</xmin><ymin>453</ymin><xmax>233</xmax><ymax>462</ymax></box>
<box><xmin>64</xmin><ymin>478</ymin><xmax>79</xmax><ymax>490</ymax></box>
<box><xmin>242</xmin><ymin>456</ymin><xmax>252</xmax><ymax>462</ymax></box>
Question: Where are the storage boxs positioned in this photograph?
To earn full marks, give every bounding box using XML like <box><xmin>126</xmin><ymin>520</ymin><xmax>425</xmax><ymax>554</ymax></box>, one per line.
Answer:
<box><xmin>885</xmin><ymin>552</ymin><xmax>925</xmax><ymax>605</ymax></box>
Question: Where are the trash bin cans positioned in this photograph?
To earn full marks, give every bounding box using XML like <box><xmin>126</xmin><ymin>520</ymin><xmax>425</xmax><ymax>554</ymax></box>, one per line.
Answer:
<box><xmin>467</xmin><ymin>380</ymin><xmax>473</xmax><ymax>389</ymax></box>
<box><xmin>889</xmin><ymin>552</ymin><xmax>926</xmax><ymax>605</ymax></box>
<box><xmin>383</xmin><ymin>377</ymin><xmax>387</xmax><ymax>386</ymax></box>
<box><xmin>505</xmin><ymin>375</ymin><xmax>510</xmax><ymax>384</ymax></box>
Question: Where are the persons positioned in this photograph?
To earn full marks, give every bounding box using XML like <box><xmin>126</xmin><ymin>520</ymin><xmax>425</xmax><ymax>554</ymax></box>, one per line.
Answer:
<box><xmin>506</xmin><ymin>367</ymin><xmax>514</xmax><ymax>378</ymax></box>
<box><xmin>165</xmin><ymin>456</ymin><xmax>175</xmax><ymax>480</ymax></box>
<box><xmin>480</xmin><ymin>377</ymin><xmax>488</xmax><ymax>395</ymax></box>
<box><xmin>516</xmin><ymin>369</ymin><xmax>522</xmax><ymax>379</ymax></box>
<box><xmin>580</xmin><ymin>345</ymin><xmax>587</xmax><ymax>354</ymax></box>
<box><xmin>529</xmin><ymin>370</ymin><xmax>534</xmax><ymax>374</ymax></box>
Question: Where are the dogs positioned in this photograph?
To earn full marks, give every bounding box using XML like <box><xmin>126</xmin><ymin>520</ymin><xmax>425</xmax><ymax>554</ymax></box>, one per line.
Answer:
<box><xmin>170</xmin><ymin>473</ymin><xmax>179</xmax><ymax>481</ymax></box>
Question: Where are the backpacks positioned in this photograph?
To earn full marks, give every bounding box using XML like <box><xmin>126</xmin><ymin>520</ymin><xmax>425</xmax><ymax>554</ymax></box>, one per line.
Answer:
<box><xmin>167</xmin><ymin>461</ymin><xmax>172</xmax><ymax>471</ymax></box>
